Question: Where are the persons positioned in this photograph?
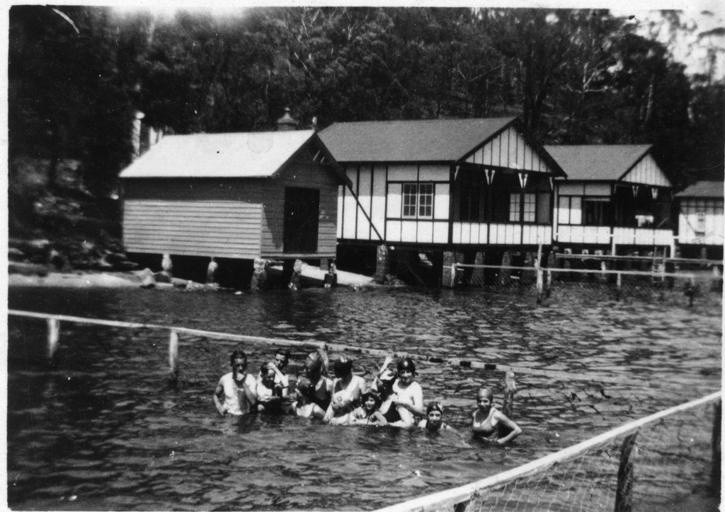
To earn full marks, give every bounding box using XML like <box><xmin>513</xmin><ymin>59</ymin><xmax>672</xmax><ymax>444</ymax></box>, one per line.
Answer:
<box><xmin>472</xmin><ymin>388</ymin><xmax>523</xmax><ymax>446</ymax></box>
<box><xmin>416</xmin><ymin>403</ymin><xmax>463</xmax><ymax>440</ymax></box>
<box><xmin>213</xmin><ymin>343</ymin><xmax>423</xmax><ymax>429</ymax></box>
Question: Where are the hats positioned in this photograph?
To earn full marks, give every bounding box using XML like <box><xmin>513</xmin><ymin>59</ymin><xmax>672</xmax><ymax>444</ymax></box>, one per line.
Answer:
<box><xmin>397</xmin><ymin>358</ymin><xmax>414</xmax><ymax>372</ymax></box>
<box><xmin>426</xmin><ymin>402</ymin><xmax>442</xmax><ymax>415</ymax></box>
<box><xmin>477</xmin><ymin>386</ymin><xmax>494</xmax><ymax>401</ymax></box>
